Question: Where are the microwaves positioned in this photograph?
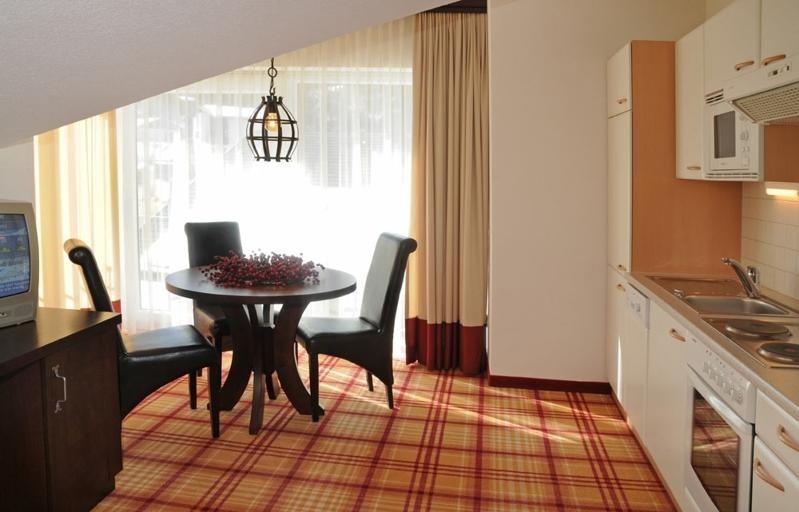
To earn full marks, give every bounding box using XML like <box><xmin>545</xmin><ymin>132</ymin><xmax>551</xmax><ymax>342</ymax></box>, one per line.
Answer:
<box><xmin>703</xmin><ymin>89</ymin><xmax>764</xmax><ymax>182</ymax></box>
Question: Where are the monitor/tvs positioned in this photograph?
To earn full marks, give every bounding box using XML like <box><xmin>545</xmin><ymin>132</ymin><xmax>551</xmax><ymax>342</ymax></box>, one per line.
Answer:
<box><xmin>1</xmin><ymin>200</ymin><xmax>40</xmax><ymax>328</ymax></box>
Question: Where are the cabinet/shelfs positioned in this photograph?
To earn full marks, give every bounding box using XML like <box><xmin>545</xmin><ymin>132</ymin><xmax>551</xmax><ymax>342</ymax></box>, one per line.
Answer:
<box><xmin>673</xmin><ymin>26</ymin><xmax>705</xmax><ymax>180</ymax></box>
<box><xmin>606</xmin><ymin>45</ymin><xmax>631</xmax><ymax>421</ymax></box>
<box><xmin>706</xmin><ymin>1</ymin><xmax>799</xmax><ymax>78</ymax></box>
<box><xmin>630</xmin><ymin>286</ymin><xmax>688</xmax><ymax>512</ymax></box>
<box><xmin>752</xmin><ymin>390</ymin><xmax>799</xmax><ymax>511</ymax></box>
<box><xmin>0</xmin><ymin>325</ymin><xmax>124</xmax><ymax>510</ymax></box>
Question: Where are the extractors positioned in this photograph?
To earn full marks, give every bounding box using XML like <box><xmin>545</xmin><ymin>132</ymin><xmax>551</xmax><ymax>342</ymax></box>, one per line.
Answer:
<box><xmin>722</xmin><ymin>54</ymin><xmax>799</xmax><ymax>125</ymax></box>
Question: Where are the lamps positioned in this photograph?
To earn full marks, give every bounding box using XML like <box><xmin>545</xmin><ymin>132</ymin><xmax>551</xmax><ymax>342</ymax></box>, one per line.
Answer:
<box><xmin>246</xmin><ymin>58</ymin><xmax>301</xmax><ymax>163</ymax></box>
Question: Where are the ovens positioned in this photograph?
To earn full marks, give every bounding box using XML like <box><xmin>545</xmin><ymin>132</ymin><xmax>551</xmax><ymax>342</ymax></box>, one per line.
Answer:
<box><xmin>682</xmin><ymin>331</ymin><xmax>757</xmax><ymax>512</ymax></box>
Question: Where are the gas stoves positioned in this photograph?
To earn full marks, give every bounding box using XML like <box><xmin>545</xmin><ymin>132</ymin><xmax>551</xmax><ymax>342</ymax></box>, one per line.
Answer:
<box><xmin>700</xmin><ymin>316</ymin><xmax>799</xmax><ymax>371</ymax></box>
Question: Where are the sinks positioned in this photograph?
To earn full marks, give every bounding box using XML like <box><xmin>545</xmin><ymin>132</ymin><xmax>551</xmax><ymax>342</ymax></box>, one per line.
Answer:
<box><xmin>682</xmin><ymin>293</ymin><xmax>798</xmax><ymax>325</ymax></box>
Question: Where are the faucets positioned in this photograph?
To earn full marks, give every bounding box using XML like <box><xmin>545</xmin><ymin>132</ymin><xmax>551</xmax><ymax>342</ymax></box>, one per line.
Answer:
<box><xmin>720</xmin><ymin>256</ymin><xmax>761</xmax><ymax>299</ymax></box>
